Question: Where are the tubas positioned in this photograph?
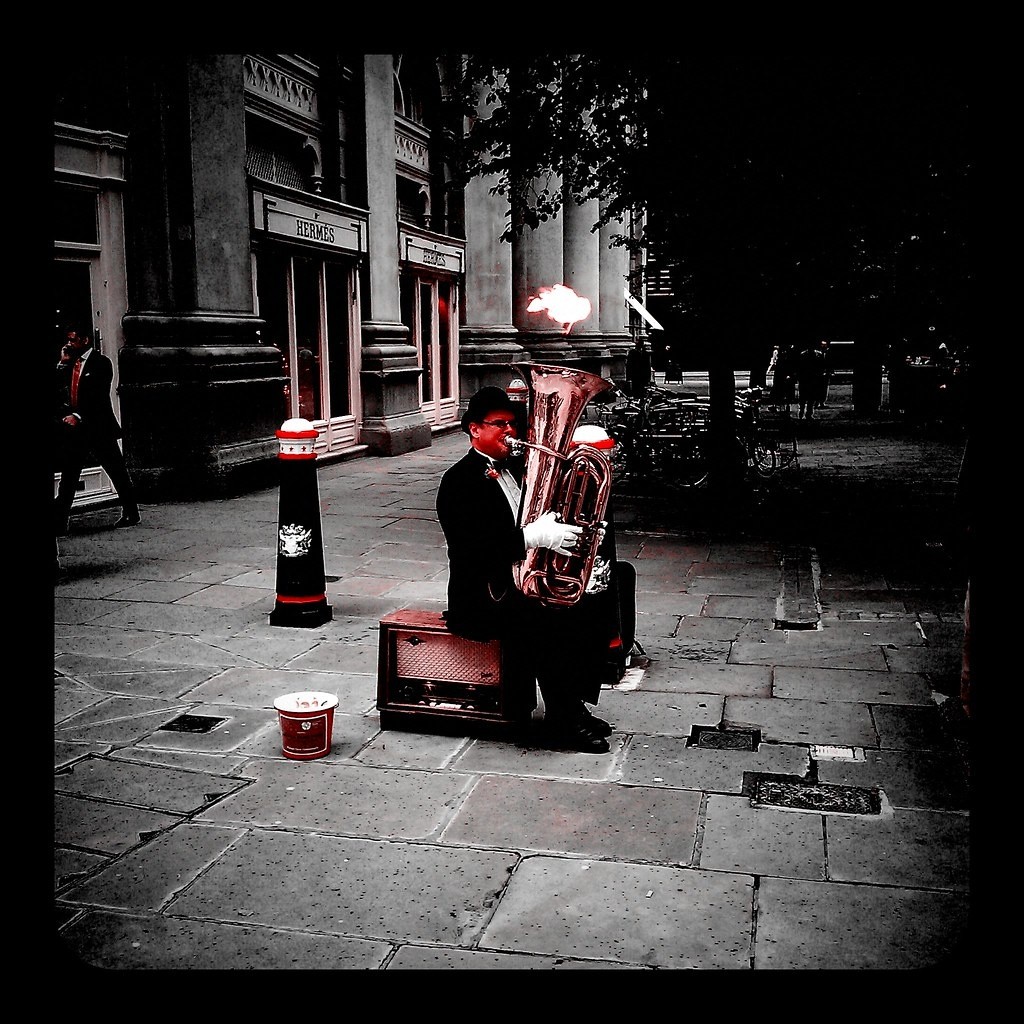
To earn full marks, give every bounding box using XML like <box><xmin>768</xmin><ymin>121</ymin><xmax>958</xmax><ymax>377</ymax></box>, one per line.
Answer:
<box><xmin>503</xmin><ymin>360</ymin><xmax>616</xmax><ymax>606</ymax></box>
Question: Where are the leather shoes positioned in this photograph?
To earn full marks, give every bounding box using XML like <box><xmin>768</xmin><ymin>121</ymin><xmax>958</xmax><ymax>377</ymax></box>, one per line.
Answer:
<box><xmin>570</xmin><ymin>699</ymin><xmax>612</xmax><ymax>736</ymax></box>
<box><xmin>543</xmin><ymin>717</ymin><xmax>609</xmax><ymax>753</ymax></box>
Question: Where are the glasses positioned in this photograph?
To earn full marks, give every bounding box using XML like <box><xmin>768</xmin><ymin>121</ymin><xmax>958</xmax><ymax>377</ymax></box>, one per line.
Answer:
<box><xmin>484</xmin><ymin>420</ymin><xmax>517</xmax><ymax>428</ymax></box>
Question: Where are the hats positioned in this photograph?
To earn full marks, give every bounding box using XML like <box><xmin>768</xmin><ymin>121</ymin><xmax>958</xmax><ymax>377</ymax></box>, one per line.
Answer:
<box><xmin>461</xmin><ymin>386</ymin><xmax>527</xmax><ymax>435</ymax></box>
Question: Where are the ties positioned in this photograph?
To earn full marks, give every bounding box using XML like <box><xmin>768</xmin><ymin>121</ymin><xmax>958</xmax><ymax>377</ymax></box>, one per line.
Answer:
<box><xmin>71</xmin><ymin>358</ymin><xmax>82</xmax><ymax>406</ymax></box>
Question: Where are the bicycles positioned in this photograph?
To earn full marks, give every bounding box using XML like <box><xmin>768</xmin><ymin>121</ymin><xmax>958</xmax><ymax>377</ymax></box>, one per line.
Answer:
<box><xmin>593</xmin><ymin>378</ymin><xmax>799</xmax><ymax>491</ymax></box>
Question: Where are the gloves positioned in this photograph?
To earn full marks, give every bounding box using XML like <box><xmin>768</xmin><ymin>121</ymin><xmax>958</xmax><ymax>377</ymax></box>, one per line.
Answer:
<box><xmin>523</xmin><ymin>511</ymin><xmax>583</xmax><ymax>557</ymax></box>
<box><xmin>580</xmin><ymin>513</ymin><xmax>608</xmax><ymax>545</ymax></box>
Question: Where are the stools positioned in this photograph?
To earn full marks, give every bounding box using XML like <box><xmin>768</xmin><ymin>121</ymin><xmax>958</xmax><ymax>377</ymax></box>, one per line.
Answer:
<box><xmin>377</xmin><ymin>609</ymin><xmax>538</xmax><ymax>738</ymax></box>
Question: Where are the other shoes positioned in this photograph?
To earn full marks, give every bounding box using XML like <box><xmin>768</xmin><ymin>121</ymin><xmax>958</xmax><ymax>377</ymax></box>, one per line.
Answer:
<box><xmin>115</xmin><ymin>516</ymin><xmax>141</xmax><ymax>528</ymax></box>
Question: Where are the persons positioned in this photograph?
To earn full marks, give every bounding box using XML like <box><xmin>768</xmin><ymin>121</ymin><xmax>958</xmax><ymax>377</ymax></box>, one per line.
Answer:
<box><xmin>435</xmin><ymin>386</ymin><xmax>613</xmax><ymax>755</ymax></box>
<box><xmin>626</xmin><ymin>338</ymin><xmax>652</xmax><ymax>398</ymax></box>
<box><xmin>51</xmin><ymin>325</ymin><xmax>141</xmax><ymax>536</ymax></box>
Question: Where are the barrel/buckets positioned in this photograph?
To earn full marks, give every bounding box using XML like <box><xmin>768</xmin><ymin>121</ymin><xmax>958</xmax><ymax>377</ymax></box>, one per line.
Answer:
<box><xmin>274</xmin><ymin>692</ymin><xmax>340</xmax><ymax>760</ymax></box>
